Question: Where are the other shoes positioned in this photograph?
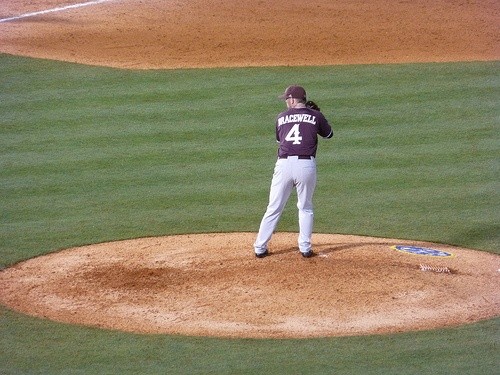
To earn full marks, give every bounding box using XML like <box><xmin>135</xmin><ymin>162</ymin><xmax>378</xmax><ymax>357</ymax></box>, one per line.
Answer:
<box><xmin>255</xmin><ymin>249</ymin><xmax>268</xmax><ymax>258</ymax></box>
<box><xmin>301</xmin><ymin>250</ymin><xmax>313</xmax><ymax>257</ymax></box>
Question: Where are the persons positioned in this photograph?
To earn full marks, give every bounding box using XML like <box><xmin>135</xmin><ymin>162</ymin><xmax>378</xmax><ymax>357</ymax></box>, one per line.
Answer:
<box><xmin>253</xmin><ymin>85</ymin><xmax>333</xmax><ymax>258</ymax></box>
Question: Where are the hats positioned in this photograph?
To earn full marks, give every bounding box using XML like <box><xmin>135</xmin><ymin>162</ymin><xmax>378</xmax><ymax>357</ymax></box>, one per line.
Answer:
<box><xmin>278</xmin><ymin>85</ymin><xmax>306</xmax><ymax>99</ymax></box>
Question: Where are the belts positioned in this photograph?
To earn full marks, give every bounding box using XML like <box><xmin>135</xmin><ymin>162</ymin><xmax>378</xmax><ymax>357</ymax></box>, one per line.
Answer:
<box><xmin>277</xmin><ymin>155</ymin><xmax>315</xmax><ymax>159</ymax></box>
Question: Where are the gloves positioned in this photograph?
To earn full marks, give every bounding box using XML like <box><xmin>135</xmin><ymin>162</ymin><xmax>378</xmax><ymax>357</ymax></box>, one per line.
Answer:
<box><xmin>306</xmin><ymin>101</ymin><xmax>320</xmax><ymax>111</ymax></box>
